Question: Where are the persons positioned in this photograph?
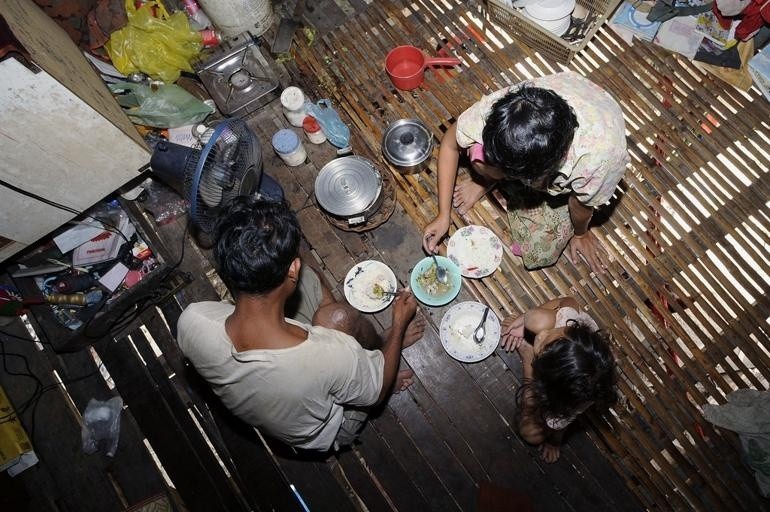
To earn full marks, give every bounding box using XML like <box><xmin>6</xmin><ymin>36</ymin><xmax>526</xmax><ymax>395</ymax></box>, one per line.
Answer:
<box><xmin>500</xmin><ymin>295</ymin><xmax>619</xmax><ymax>466</ymax></box>
<box><xmin>175</xmin><ymin>189</ymin><xmax>426</xmax><ymax>461</ymax></box>
<box><xmin>419</xmin><ymin>70</ymin><xmax>630</xmax><ymax>279</ymax></box>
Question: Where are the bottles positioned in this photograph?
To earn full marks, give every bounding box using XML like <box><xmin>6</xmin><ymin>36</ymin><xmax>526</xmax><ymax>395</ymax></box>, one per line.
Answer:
<box><xmin>280</xmin><ymin>85</ymin><xmax>313</xmax><ymax>128</ymax></box>
<box><xmin>271</xmin><ymin>129</ymin><xmax>308</xmax><ymax>167</ymax></box>
<box><xmin>303</xmin><ymin>116</ymin><xmax>326</xmax><ymax>145</ymax></box>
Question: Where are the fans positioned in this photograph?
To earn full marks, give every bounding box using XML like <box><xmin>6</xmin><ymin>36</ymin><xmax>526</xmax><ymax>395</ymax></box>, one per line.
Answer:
<box><xmin>144</xmin><ymin>116</ymin><xmax>286</xmax><ymax>238</ymax></box>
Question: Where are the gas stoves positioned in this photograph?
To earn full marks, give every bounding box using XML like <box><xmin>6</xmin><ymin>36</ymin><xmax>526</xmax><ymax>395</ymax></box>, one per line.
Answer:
<box><xmin>191</xmin><ymin>30</ymin><xmax>282</xmax><ymax>121</ymax></box>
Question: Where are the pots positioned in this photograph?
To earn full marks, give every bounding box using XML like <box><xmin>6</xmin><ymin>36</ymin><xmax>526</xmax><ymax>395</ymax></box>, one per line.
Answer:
<box><xmin>381</xmin><ymin>118</ymin><xmax>434</xmax><ymax>176</ymax></box>
<box><xmin>314</xmin><ymin>150</ymin><xmax>384</xmax><ymax>226</ymax></box>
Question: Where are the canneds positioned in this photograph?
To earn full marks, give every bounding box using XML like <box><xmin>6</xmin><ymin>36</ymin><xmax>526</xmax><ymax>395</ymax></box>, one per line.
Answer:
<box><xmin>199</xmin><ymin>30</ymin><xmax>222</xmax><ymax>48</ymax></box>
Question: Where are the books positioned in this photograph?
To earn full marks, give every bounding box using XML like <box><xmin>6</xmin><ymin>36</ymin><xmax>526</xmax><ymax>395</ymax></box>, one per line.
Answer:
<box><xmin>610</xmin><ymin>1</ymin><xmax>770</xmax><ymax>102</ymax></box>
<box><xmin>70</xmin><ymin>210</ymin><xmax>129</xmax><ymax>268</ymax></box>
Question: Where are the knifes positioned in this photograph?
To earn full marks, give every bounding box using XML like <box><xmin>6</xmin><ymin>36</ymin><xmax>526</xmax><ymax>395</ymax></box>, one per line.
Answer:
<box><xmin>270</xmin><ymin>0</ymin><xmax>306</xmax><ymax>54</ymax></box>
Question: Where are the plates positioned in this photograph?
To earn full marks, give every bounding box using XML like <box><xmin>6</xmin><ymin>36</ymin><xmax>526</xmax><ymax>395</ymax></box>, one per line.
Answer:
<box><xmin>439</xmin><ymin>300</ymin><xmax>501</xmax><ymax>364</ymax></box>
<box><xmin>343</xmin><ymin>259</ymin><xmax>398</xmax><ymax>314</ymax></box>
<box><xmin>446</xmin><ymin>224</ymin><xmax>504</xmax><ymax>279</ymax></box>
<box><xmin>492</xmin><ymin>0</ymin><xmax>575</xmax><ymax>39</ymax></box>
<box><xmin>410</xmin><ymin>254</ymin><xmax>462</xmax><ymax>308</ymax></box>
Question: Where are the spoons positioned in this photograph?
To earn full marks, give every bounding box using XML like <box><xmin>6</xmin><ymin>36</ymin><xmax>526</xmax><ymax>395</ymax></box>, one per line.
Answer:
<box><xmin>431</xmin><ymin>251</ymin><xmax>448</xmax><ymax>285</ymax></box>
<box><xmin>473</xmin><ymin>307</ymin><xmax>489</xmax><ymax>344</ymax></box>
